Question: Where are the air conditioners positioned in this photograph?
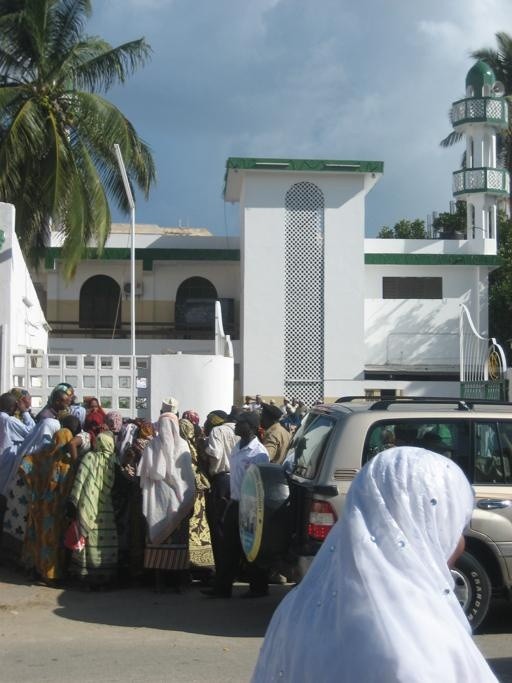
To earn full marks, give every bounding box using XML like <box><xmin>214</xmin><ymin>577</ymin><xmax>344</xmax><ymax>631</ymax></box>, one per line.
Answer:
<box><xmin>121</xmin><ymin>280</ymin><xmax>143</xmax><ymax>295</ymax></box>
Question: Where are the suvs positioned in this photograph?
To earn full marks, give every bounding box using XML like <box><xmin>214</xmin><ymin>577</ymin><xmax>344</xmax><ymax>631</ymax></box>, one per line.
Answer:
<box><xmin>239</xmin><ymin>396</ymin><xmax>512</xmax><ymax>634</ymax></box>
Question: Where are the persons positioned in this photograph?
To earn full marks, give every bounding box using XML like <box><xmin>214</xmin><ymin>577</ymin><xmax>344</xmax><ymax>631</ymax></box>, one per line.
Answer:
<box><xmin>1</xmin><ymin>381</ymin><xmax>325</xmax><ymax>600</ymax></box>
<box><xmin>246</xmin><ymin>444</ymin><xmax>504</xmax><ymax>683</ymax></box>
<box><xmin>366</xmin><ymin>418</ymin><xmax>511</xmax><ymax>483</ymax></box>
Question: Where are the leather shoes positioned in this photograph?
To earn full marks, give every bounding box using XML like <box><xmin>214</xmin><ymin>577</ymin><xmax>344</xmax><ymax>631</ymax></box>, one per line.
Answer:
<box><xmin>199</xmin><ymin>587</ymin><xmax>233</xmax><ymax>598</ymax></box>
<box><xmin>241</xmin><ymin>590</ymin><xmax>269</xmax><ymax>599</ymax></box>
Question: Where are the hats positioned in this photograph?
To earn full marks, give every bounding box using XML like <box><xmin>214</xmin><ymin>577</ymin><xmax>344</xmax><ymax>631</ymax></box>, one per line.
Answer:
<box><xmin>231</xmin><ymin>410</ymin><xmax>260</xmax><ymax>427</ymax></box>
<box><xmin>263</xmin><ymin>403</ymin><xmax>282</xmax><ymax>419</ymax></box>
